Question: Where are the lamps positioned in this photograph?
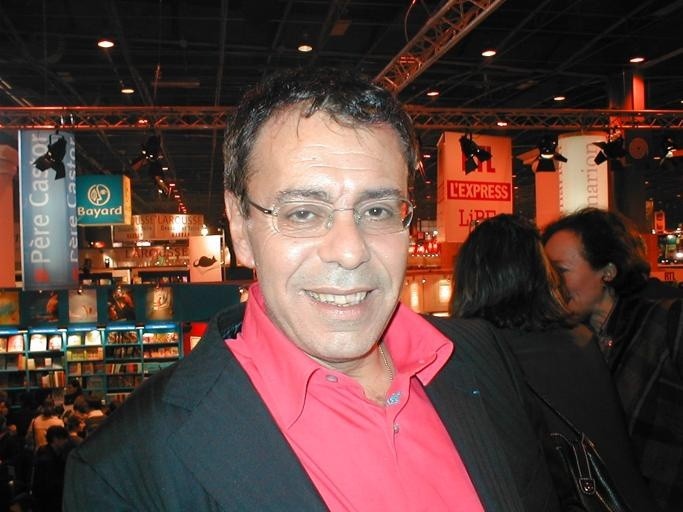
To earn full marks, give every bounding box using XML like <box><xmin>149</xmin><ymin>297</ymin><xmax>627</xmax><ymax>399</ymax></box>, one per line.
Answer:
<box><xmin>33</xmin><ymin>129</ymin><xmax>66</xmax><ymax>179</ymax></box>
<box><xmin>130</xmin><ymin>127</ymin><xmax>165</xmax><ymax>177</ymax></box>
<box><xmin>532</xmin><ymin>130</ymin><xmax>567</xmax><ymax>171</ymax></box>
<box><xmin>591</xmin><ymin>128</ymin><xmax>628</xmax><ymax>171</ymax></box>
<box><xmin>648</xmin><ymin>129</ymin><xmax>675</xmax><ymax>166</ymax></box>
<box><xmin>458</xmin><ymin>128</ymin><xmax>493</xmax><ymax>174</ymax></box>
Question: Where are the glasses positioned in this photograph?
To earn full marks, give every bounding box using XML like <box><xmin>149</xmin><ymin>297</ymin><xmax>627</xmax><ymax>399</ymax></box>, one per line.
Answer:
<box><xmin>244</xmin><ymin>190</ymin><xmax>415</xmax><ymax>238</ymax></box>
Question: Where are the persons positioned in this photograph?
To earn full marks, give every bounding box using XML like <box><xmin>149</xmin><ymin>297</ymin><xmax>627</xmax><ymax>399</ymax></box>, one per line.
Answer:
<box><xmin>32</xmin><ymin>292</ymin><xmax>61</xmax><ymax>323</ymax></box>
<box><xmin>0</xmin><ymin>380</ymin><xmax>123</xmax><ymax>512</ymax></box>
<box><xmin>107</xmin><ymin>285</ymin><xmax>136</xmax><ymax>323</ymax></box>
<box><xmin>59</xmin><ymin>63</ymin><xmax>561</xmax><ymax>512</ymax></box>
<box><xmin>534</xmin><ymin>204</ymin><xmax>683</xmax><ymax>512</ymax></box>
<box><xmin>447</xmin><ymin>213</ymin><xmax>658</xmax><ymax>511</ymax></box>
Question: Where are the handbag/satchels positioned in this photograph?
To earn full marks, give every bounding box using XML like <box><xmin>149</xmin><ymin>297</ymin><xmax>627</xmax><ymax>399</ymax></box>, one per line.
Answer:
<box><xmin>545</xmin><ymin>431</ymin><xmax>633</xmax><ymax>512</ymax></box>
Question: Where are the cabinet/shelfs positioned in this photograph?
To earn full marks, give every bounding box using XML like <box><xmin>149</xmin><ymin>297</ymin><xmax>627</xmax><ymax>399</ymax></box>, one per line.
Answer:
<box><xmin>0</xmin><ymin>322</ymin><xmax>185</xmax><ymax>410</ymax></box>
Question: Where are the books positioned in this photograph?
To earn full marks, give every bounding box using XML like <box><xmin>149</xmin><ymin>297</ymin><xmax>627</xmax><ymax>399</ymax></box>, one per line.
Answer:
<box><xmin>0</xmin><ymin>329</ymin><xmax>179</xmax><ymax>407</ymax></box>
